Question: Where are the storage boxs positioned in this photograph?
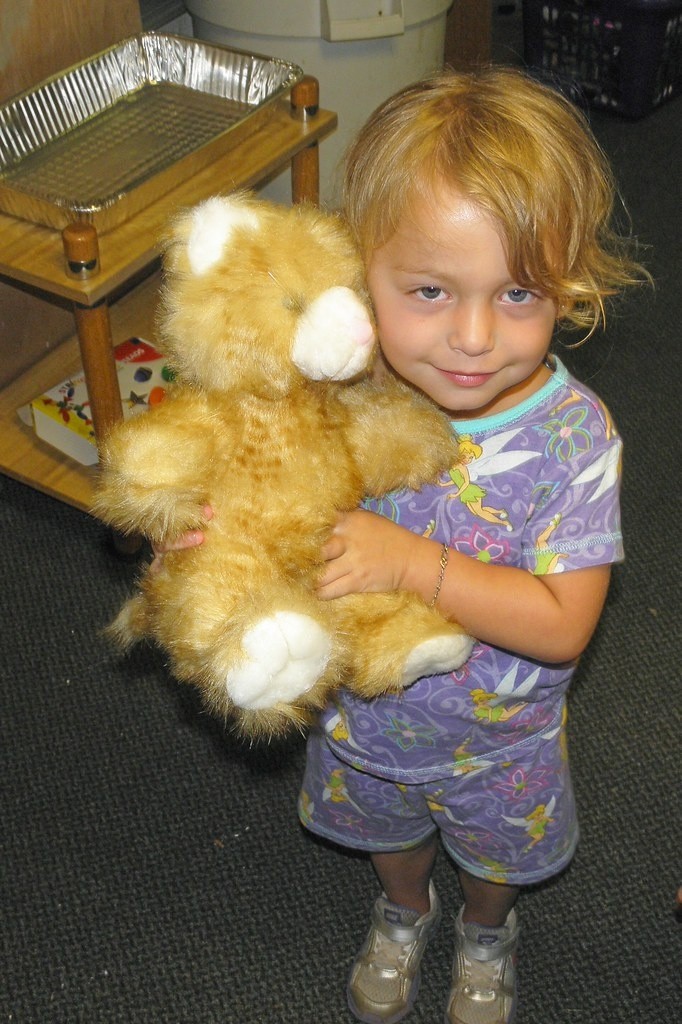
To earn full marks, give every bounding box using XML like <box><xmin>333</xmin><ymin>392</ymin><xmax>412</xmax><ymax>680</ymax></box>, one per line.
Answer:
<box><xmin>521</xmin><ymin>0</ymin><xmax>682</xmax><ymax>123</ymax></box>
<box><xmin>16</xmin><ymin>336</ymin><xmax>179</xmax><ymax>467</ymax></box>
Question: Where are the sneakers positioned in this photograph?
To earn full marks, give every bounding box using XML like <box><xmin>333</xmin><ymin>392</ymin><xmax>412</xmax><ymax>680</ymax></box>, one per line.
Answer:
<box><xmin>346</xmin><ymin>877</ymin><xmax>443</xmax><ymax>1024</ymax></box>
<box><xmin>444</xmin><ymin>902</ymin><xmax>522</xmax><ymax>1024</ymax></box>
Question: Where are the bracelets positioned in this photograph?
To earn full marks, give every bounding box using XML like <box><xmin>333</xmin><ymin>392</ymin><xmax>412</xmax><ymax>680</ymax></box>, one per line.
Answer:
<box><xmin>430</xmin><ymin>541</ymin><xmax>447</xmax><ymax>607</ymax></box>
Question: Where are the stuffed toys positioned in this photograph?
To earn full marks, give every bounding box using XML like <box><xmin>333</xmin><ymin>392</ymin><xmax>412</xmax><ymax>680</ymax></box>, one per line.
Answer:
<box><xmin>90</xmin><ymin>194</ymin><xmax>473</xmax><ymax>739</ymax></box>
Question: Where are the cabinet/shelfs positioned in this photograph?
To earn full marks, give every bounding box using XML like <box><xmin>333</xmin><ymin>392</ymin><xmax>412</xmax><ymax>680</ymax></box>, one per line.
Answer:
<box><xmin>0</xmin><ymin>74</ymin><xmax>337</xmax><ymax>565</ymax></box>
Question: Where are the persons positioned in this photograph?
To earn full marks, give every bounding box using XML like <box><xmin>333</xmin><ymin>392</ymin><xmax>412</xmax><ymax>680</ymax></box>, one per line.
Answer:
<box><xmin>146</xmin><ymin>68</ymin><xmax>652</xmax><ymax>1024</ymax></box>
